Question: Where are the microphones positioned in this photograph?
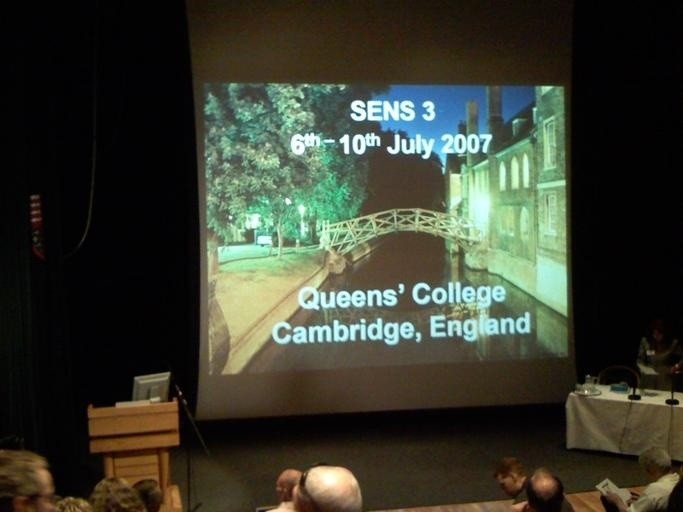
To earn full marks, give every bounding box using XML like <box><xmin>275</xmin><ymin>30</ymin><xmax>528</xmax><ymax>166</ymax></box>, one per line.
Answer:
<box><xmin>175</xmin><ymin>383</ymin><xmax>187</xmax><ymax>406</ymax></box>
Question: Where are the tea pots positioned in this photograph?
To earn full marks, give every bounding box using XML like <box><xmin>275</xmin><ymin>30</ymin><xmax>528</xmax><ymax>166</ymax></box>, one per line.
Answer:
<box><xmin>583</xmin><ymin>374</ymin><xmax>599</xmax><ymax>393</ymax></box>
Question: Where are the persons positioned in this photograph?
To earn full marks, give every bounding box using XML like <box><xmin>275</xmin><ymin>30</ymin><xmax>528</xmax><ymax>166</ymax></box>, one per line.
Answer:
<box><xmin>636</xmin><ymin>320</ymin><xmax>683</xmax><ymax>391</ymax></box>
<box><xmin>0</xmin><ymin>450</ymin><xmax>161</xmax><ymax>512</ymax></box>
<box><xmin>265</xmin><ymin>463</ymin><xmax>363</xmax><ymax>512</ymax></box>
<box><xmin>492</xmin><ymin>446</ymin><xmax>683</xmax><ymax>512</ymax></box>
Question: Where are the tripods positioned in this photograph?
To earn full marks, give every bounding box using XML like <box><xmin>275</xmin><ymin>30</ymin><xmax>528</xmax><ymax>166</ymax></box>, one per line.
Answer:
<box><xmin>181</xmin><ymin>404</ymin><xmax>211</xmax><ymax>512</ymax></box>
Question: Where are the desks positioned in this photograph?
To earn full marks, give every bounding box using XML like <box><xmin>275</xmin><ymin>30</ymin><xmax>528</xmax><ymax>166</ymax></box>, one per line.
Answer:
<box><xmin>569</xmin><ymin>382</ymin><xmax>683</xmax><ymax>462</ymax></box>
<box><xmin>354</xmin><ymin>485</ymin><xmax>646</xmax><ymax>512</ymax></box>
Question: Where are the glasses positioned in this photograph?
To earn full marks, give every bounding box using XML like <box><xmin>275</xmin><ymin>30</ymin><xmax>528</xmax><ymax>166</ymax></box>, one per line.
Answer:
<box><xmin>299</xmin><ymin>461</ymin><xmax>327</xmax><ymax>512</ymax></box>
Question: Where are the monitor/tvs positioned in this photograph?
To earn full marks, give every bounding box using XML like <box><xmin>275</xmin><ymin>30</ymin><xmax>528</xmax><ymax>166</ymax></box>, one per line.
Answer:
<box><xmin>132</xmin><ymin>371</ymin><xmax>171</xmax><ymax>403</ymax></box>
